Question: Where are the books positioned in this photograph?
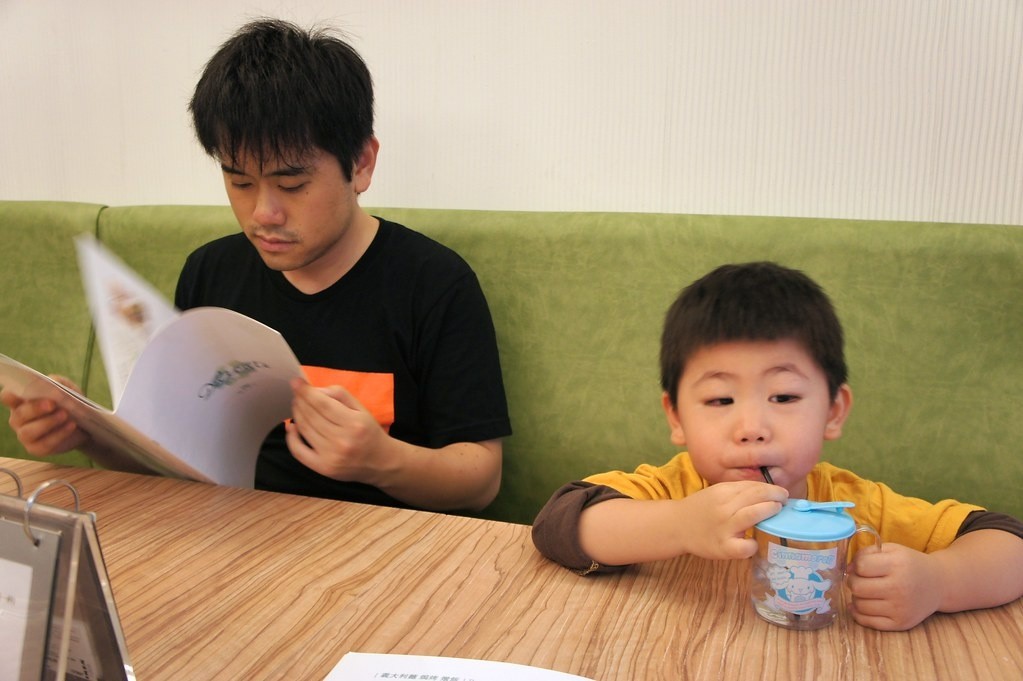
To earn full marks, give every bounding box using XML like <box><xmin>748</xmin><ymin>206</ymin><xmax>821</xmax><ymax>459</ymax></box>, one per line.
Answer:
<box><xmin>0</xmin><ymin>228</ymin><xmax>311</xmax><ymax>491</ymax></box>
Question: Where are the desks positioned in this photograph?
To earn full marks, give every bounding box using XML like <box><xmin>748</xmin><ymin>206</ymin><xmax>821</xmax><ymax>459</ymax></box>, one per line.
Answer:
<box><xmin>0</xmin><ymin>457</ymin><xmax>1023</xmax><ymax>681</ymax></box>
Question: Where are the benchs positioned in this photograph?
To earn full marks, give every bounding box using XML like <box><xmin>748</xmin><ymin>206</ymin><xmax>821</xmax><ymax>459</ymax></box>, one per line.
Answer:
<box><xmin>85</xmin><ymin>205</ymin><xmax>1023</xmax><ymax>523</ymax></box>
<box><xmin>0</xmin><ymin>199</ymin><xmax>109</xmax><ymax>468</ymax></box>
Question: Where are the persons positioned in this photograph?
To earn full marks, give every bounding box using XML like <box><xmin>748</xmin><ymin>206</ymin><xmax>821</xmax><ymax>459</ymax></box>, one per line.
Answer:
<box><xmin>0</xmin><ymin>19</ymin><xmax>513</xmax><ymax>514</ymax></box>
<box><xmin>531</xmin><ymin>261</ymin><xmax>1023</xmax><ymax>633</ymax></box>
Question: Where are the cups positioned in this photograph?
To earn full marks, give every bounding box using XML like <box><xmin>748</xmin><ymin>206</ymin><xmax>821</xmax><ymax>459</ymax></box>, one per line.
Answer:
<box><xmin>746</xmin><ymin>497</ymin><xmax>884</xmax><ymax>631</ymax></box>
